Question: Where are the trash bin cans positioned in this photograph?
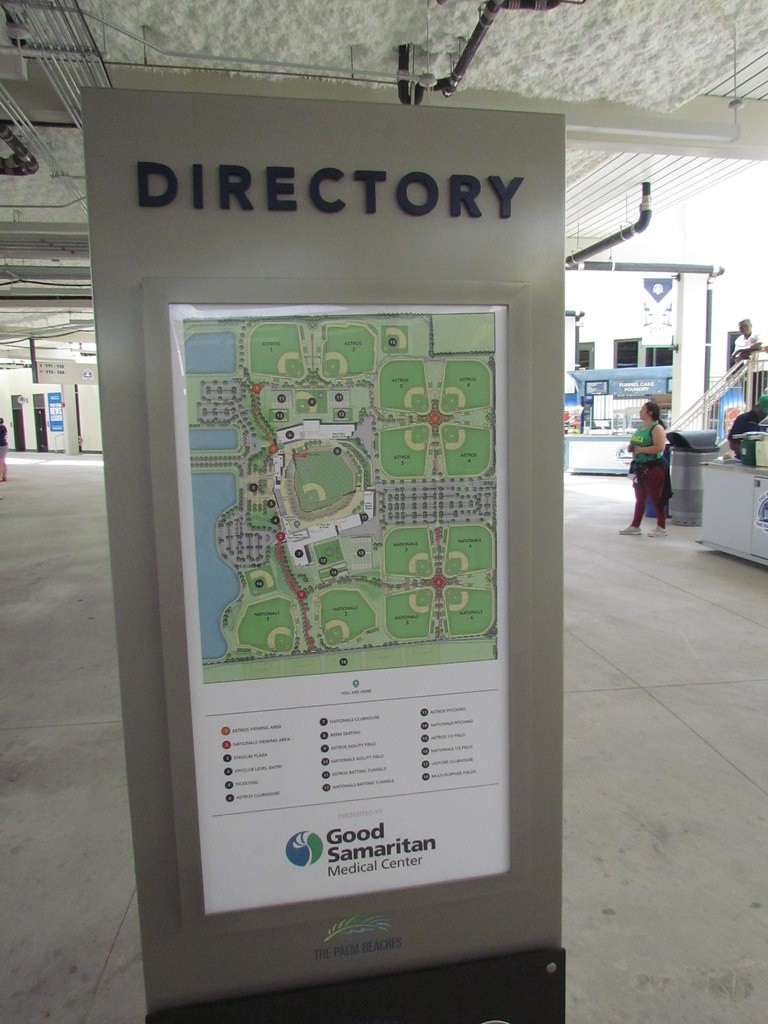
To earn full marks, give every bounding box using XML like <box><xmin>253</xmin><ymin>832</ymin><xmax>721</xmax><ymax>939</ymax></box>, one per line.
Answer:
<box><xmin>645</xmin><ymin>444</ymin><xmax>671</xmax><ymax>518</ymax></box>
<box><xmin>664</xmin><ymin>429</ymin><xmax>721</xmax><ymax>528</ymax></box>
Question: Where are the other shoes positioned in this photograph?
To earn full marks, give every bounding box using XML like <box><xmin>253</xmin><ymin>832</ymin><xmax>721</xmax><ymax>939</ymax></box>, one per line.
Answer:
<box><xmin>3</xmin><ymin>478</ymin><xmax>7</xmax><ymax>481</ymax></box>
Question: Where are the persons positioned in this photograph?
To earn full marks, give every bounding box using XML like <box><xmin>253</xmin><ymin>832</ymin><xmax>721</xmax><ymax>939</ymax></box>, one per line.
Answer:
<box><xmin>726</xmin><ymin>393</ymin><xmax>768</xmax><ymax>457</ymax></box>
<box><xmin>0</xmin><ymin>418</ymin><xmax>9</xmax><ymax>482</ymax></box>
<box><xmin>730</xmin><ymin>317</ymin><xmax>766</xmax><ymax>409</ymax></box>
<box><xmin>619</xmin><ymin>402</ymin><xmax>671</xmax><ymax>537</ymax></box>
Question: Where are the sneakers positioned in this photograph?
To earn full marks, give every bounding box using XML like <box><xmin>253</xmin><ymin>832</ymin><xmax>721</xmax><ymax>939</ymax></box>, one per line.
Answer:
<box><xmin>648</xmin><ymin>526</ymin><xmax>667</xmax><ymax>537</ymax></box>
<box><xmin>620</xmin><ymin>526</ymin><xmax>641</xmax><ymax>535</ymax></box>
